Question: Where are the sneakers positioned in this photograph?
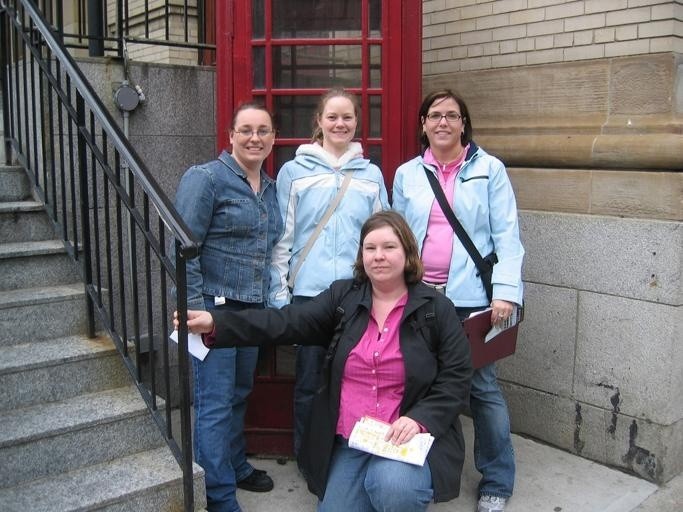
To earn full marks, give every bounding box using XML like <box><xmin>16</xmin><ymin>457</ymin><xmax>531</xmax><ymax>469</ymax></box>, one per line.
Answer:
<box><xmin>237</xmin><ymin>468</ymin><xmax>273</xmax><ymax>492</ymax></box>
<box><xmin>476</xmin><ymin>494</ymin><xmax>507</xmax><ymax>512</ymax></box>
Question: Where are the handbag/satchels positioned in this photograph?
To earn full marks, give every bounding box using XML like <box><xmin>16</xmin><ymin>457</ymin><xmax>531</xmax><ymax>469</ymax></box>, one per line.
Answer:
<box><xmin>477</xmin><ymin>252</ymin><xmax>498</xmax><ymax>304</ymax></box>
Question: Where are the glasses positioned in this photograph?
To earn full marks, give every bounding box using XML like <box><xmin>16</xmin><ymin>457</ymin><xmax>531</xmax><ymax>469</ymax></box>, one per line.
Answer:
<box><xmin>232</xmin><ymin>126</ymin><xmax>276</xmax><ymax>137</ymax></box>
<box><xmin>424</xmin><ymin>111</ymin><xmax>463</xmax><ymax>122</ymax></box>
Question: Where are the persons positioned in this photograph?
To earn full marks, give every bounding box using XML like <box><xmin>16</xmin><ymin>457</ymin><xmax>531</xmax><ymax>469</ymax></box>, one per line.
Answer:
<box><xmin>262</xmin><ymin>84</ymin><xmax>392</xmax><ymax>473</ymax></box>
<box><xmin>171</xmin><ymin>210</ymin><xmax>478</xmax><ymax>511</ymax></box>
<box><xmin>167</xmin><ymin>99</ymin><xmax>285</xmax><ymax>511</ymax></box>
<box><xmin>381</xmin><ymin>86</ymin><xmax>525</xmax><ymax>512</ymax></box>
